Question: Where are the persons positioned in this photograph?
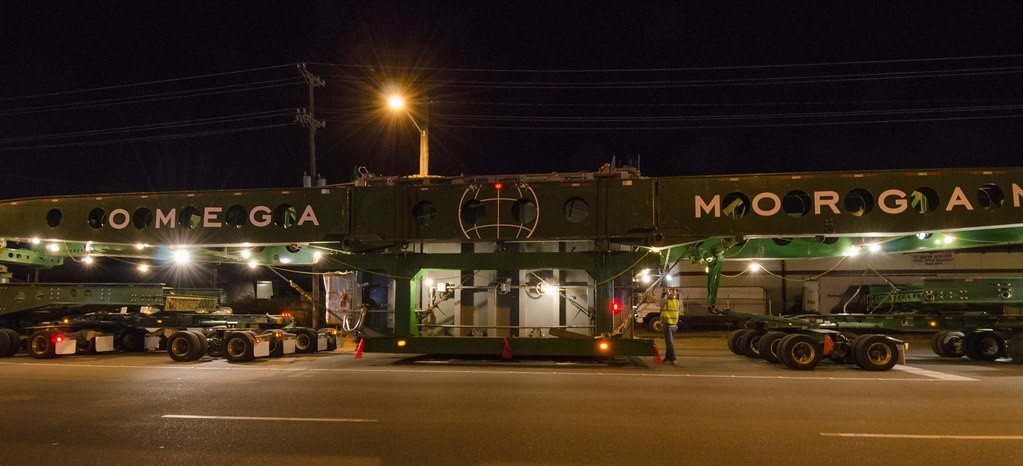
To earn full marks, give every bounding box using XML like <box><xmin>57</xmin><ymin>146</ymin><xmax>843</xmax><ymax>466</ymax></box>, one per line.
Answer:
<box><xmin>654</xmin><ymin>286</ymin><xmax>680</xmax><ymax>364</ymax></box>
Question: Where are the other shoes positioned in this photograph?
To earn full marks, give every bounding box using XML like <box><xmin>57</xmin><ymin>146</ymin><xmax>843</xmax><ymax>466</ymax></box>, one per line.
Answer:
<box><xmin>663</xmin><ymin>359</ymin><xmax>677</xmax><ymax>365</ymax></box>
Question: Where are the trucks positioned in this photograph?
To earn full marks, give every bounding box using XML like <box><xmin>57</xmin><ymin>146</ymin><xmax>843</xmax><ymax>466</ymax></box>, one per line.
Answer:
<box><xmin>634</xmin><ymin>287</ymin><xmax>766</xmax><ymax>333</ymax></box>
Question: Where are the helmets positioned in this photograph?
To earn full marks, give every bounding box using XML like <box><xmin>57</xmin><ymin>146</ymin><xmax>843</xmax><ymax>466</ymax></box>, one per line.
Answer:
<box><xmin>667</xmin><ymin>288</ymin><xmax>677</xmax><ymax>299</ymax></box>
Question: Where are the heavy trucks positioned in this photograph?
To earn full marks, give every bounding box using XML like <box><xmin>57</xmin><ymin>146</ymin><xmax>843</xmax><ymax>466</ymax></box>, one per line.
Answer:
<box><xmin>0</xmin><ymin>246</ymin><xmax>318</xmax><ymax>360</ymax></box>
<box><xmin>739</xmin><ymin>276</ymin><xmax>1023</xmax><ymax>371</ymax></box>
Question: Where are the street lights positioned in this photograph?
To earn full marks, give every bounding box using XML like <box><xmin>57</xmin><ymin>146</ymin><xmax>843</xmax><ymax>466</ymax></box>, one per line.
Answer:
<box><xmin>389</xmin><ymin>95</ymin><xmax>429</xmax><ymax>175</ymax></box>
